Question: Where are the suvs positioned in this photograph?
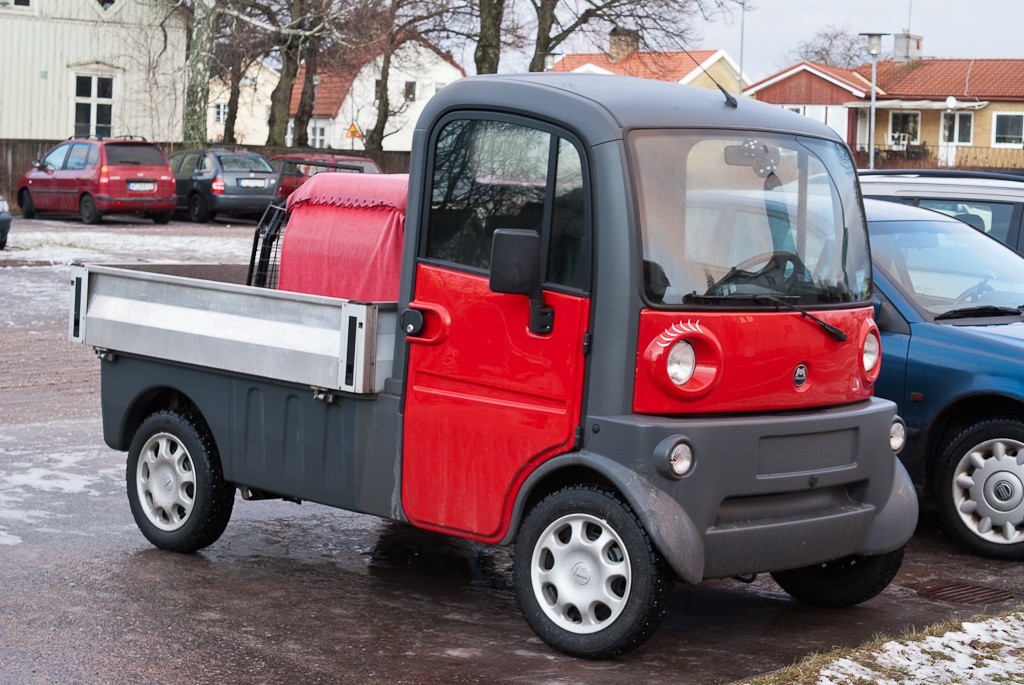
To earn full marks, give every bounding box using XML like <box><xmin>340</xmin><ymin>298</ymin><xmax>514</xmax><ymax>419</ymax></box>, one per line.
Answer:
<box><xmin>15</xmin><ymin>133</ymin><xmax>179</xmax><ymax>226</ymax></box>
<box><xmin>265</xmin><ymin>152</ymin><xmax>383</xmax><ymax>201</ymax></box>
<box><xmin>768</xmin><ymin>165</ymin><xmax>1022</xmax><ymax>262</ymax></box>
<box><xmin>167</xmin><ymin>146</ymin><xmax>286</xmax><ymax>227</ymax></box>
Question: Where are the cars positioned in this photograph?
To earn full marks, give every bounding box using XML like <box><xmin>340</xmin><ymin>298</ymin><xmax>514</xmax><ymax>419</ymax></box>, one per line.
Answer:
<box><xmin>669</xmin><ymin>179</ymin><xmax>1023</xmax><ymax>564</ymax></box>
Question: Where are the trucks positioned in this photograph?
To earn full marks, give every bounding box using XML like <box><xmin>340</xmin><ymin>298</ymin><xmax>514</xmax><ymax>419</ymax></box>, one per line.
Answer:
<box><xmin>62</xmin><ymin>71</ymin><xmax>922</xmax><ymax>665</ymax></box>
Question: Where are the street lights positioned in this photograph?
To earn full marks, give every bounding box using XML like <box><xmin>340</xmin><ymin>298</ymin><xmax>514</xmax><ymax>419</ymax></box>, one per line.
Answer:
<box><xmin>859</xmin><ymin>32</ymin><xmax>891</xmax><ymax>170</ymax></box>
